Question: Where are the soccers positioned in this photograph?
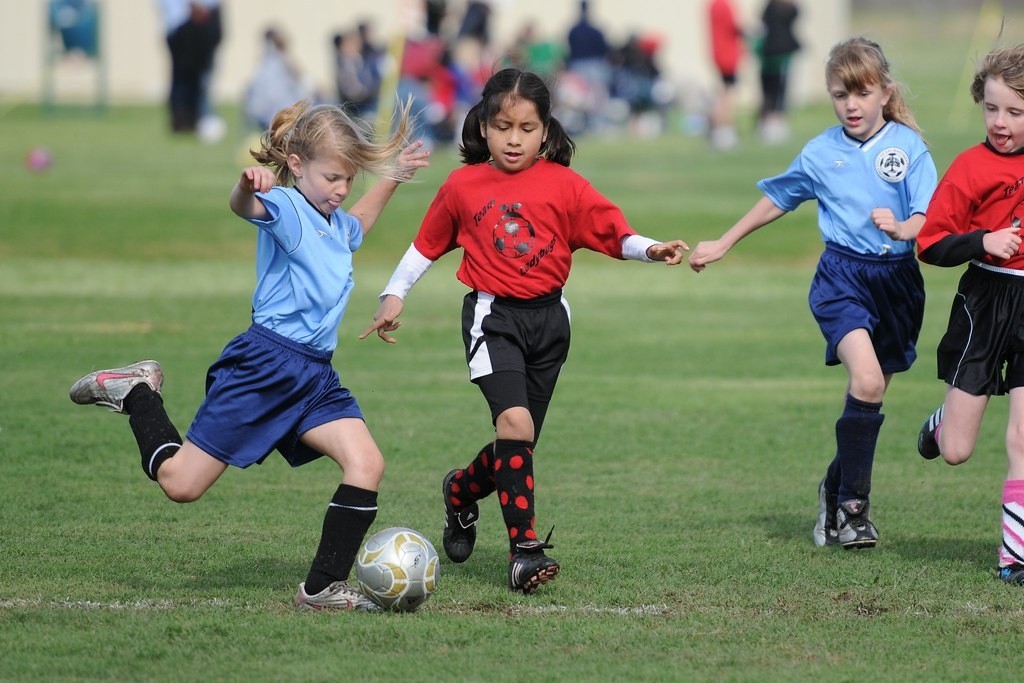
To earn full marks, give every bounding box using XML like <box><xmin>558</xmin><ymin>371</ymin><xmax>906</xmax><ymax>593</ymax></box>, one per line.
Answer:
<box><xmin>352</xmin><ymin>525</ymin><xmax>443</xmax><ymax>613</ymax></box>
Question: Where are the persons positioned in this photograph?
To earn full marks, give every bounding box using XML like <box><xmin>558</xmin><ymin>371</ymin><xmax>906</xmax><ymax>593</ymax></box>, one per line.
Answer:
<box><xmin>333</xmin><ymin>0</ymin><xmax>673</xmax><ymax>151</ymax></box>
<box><xmin>917</xmin><ymin>48</ymin><xmax>1024</xmax><ymax>587</ymax></box>
<box><xmin>704</xmin><ymin>0</ymin><xmax>745</xmax><ymax>145</ymax></box>
<box><xmin>688</xmin><ymin>37</ymin><xmax>936</xmax><ymax>547</ymax></box>
<box><xmin>241</xmin><ymin>30</ymin><xmax>323</xmax><ymax>134</ymax></box>
<box><xmin>359</xmin><ymin>67</ymin><xmax>691</xmax><ymax>595</ymax></box>
<box><xmin>162</xmin><ymin>0</ymin><xmax>228</xmax><ymax>139</ymax></box>
<box><xmin>70</xmin><ymin>95</ymin><xmax>429</xmax><ymax>610</ymax></box>
<box><xmin>748</xmin><ymin>0</ymin><xmax>801</xmax><ymax>145</ymax></box>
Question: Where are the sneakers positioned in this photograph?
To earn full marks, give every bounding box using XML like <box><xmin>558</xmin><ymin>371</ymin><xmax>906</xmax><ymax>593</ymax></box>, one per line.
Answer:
<box><xmin>69</xmin><ymin>360</ymin><xmax>164</xmax><ymax>415</ymax></box>
<box><xmin>813</xmin><ymin>474</ymin><xmax>840</xmax><ymax>546</ymax></box>
<box><xmin>837</xmin><ymin>500</ymin><xmax>879</xmax><ymax>550</ymax></box>
<box><xmin>508</xmin><ymin>524</ymin><xmax>560</xmax><ymax>596</ymax></box>
<box><xmin>442</xmin><ymin>469</ymin><xmax>479</xmax><ymax>562</ymax></box>
<box><xmin>918</xmin><ymin>403</ymin><xmax>944</xmax><ymax>460</ymax></box>
<box><xmin>997</xmin><ymin>562</ymin><xmax>1024</xmax><ymax>587</ymax></box>
<box><xmin>295</xmin><ymin>581</ymin><xmax>384</xmax><ymax>615</ymax></box>
